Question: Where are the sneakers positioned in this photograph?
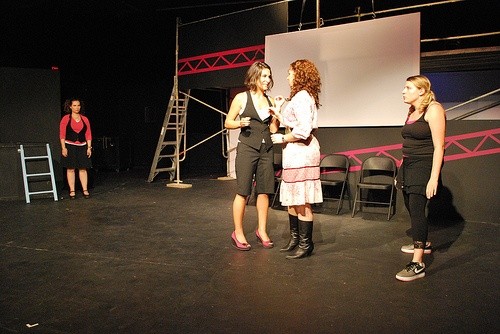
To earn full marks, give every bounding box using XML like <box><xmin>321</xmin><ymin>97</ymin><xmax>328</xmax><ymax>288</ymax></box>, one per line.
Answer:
<box><xmin>400</xmin><ymin>242</ymin><xmax>431</xmax><ymax>254</ymax></box>
<box><xmin>396</xmin><ymin>262</ymin><xmax>425</xmax><ymax>281</ymax></box>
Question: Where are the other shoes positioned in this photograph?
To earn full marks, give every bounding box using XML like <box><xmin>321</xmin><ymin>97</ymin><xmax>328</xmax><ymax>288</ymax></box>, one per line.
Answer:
<box><xmin>83</xmin><ymin>190</ymin><xmax>90</xmax><ymax>199</ymax></box>
<box><xmin>70</xmin><ymin>191</ymin><xmax>76</xmax><ymax>200</ymax></box>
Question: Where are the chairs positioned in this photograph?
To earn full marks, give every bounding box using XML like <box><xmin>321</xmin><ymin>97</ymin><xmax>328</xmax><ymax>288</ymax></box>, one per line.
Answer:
<box><xmin>246</xmin><ymin>154</ymin><xmax>283</xmax><ymax>208</ymax></box>
<box><xmin>320</xmin><ymin>154</ymin><xmax>351</xmax><ymax>215</ymax></box>
<box><xmin>350</xmin><ymin>156</ymin><xmax>396</xmax><ymax>221</ymax></box>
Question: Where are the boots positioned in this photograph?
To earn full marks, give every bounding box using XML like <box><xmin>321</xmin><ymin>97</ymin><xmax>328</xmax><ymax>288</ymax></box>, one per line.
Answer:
<box><xmin>280</xmin><ymin>213</ymin><xmax>298</xmax><ymax>253</ymax></box>
<box><xmin>285</xmin><ymin>219</ymin><xmax>313</xmax><ymax>259</ymax></box>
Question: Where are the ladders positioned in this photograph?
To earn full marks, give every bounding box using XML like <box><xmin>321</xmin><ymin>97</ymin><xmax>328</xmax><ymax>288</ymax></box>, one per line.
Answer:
<box><xmin>19</xmin><ymin>142</ymin><xmax>60</xmax><ymax>204</ymax></box>
<box><xmin>145</xmin><ymin>86</ymin><xmax>192</xmax><ymax>185</ymax></box>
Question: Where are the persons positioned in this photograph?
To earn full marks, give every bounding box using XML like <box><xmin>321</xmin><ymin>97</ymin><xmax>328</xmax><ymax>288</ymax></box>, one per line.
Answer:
<box><xmin>395</xmin><ymin>75</ymin><xmax>446</xmax><ymax>281</ymax></box>
<box><xmin>60</xmin><ymin>99</ymin><xmax>92</xmax><ymax>199</ymax></box>
<box><xmin>268</xmin><ymin>59</ymin><xmax>324</xmax><ymax>258</ymax></box>
<box><xmin>225</xmin><ymin>62</ymin><xmax>284</xmax><ymax>250</ymax></box>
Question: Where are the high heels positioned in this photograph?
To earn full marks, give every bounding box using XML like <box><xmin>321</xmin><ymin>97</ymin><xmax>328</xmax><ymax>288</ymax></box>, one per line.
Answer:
<box><xmin>231</xmin><ymin>232</ymin><xmax>251</xmax><ymax>250</ymax></box>
<box><xmin>256</xmin><ymin>229</ymin><xmax>274</xmax><ymax>248</ymax></box>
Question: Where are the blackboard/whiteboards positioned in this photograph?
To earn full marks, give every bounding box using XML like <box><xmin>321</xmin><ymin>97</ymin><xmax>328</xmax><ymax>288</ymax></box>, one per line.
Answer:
<box><xmin>263</xmin><ymin>11</ymin><xmax>422</xmax><ymax>128</ymax></box>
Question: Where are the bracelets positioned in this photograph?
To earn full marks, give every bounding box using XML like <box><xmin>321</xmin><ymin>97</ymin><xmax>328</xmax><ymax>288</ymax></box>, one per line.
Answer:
<box><xmin>87</xmin><ymin>147</ymin><xmax>92</xmax><ymax>148</ymax></box>
<box><xmin>282</xmin><ymin>135</ymin><xmax>285</xmax><ymax>143</ymax></box>
